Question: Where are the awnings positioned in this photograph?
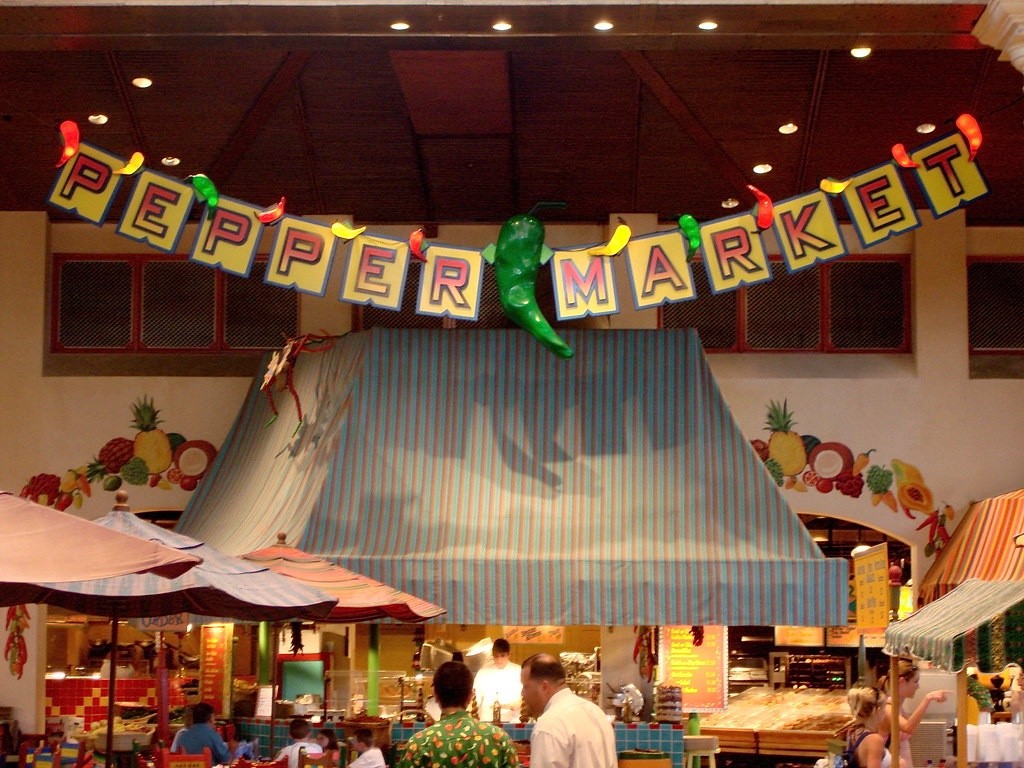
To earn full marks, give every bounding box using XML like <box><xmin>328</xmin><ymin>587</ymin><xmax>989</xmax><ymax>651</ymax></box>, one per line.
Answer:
<box><xmin>880</xmin><ymin>579</ymin><xmax>1024</xmax><ymax>768</ymax></box>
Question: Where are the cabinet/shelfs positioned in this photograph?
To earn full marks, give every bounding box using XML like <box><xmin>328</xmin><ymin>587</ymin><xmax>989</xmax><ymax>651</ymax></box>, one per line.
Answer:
<box><xmin>786</xmin><ymin>655</ymin><xmax>851</xmax><ymax>688</ymax></box>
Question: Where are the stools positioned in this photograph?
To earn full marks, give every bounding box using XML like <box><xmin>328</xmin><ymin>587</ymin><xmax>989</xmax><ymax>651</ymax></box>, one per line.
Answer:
<box><xmin>684</xmin><ymin>747</ymin><xmax>721</xmax><ymax>768</ymax></box>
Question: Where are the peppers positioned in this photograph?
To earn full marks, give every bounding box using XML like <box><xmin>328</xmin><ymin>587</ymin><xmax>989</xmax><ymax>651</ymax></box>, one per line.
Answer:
<box><xmin>688</xmin><ymin>626</ymin><xmax>704</xmax><ymax>647</ymax></box>
<box><xmin>493</xmin><ymin>212</ymin><xmax>574</xmax><ymax>360</ymax></box>
<box><xmin>289</xmin><ymin>621</ymin><xmax>304</xmax><ymax>657</ymax></box>
<box><xmin>583</xmin><ymin>112</ymin><xmax>981</xmax><ymax>262</ymax></box>
<box><xmin>54</xmin><ymin>120</ymin><xmax>367</xmax><ymax>240</ymax></box>
<box><xmin>631</xmin><ymin>625</ymin><xmax>658</xmax><ymax>682</ymax></box>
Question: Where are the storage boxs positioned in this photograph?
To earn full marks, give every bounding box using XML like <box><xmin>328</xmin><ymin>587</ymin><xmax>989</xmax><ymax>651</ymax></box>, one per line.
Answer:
<box><xmin>97</xmin><ymin>726</ymin><xmax>155</xmax><ymax>750</ymax></box>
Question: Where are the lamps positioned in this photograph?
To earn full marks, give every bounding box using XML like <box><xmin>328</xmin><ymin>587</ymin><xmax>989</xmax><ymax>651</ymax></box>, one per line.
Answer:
<box><xmin>850</xmin><ymin>526</ymin><xmax>872</xmax><ymax>558</ymax></box>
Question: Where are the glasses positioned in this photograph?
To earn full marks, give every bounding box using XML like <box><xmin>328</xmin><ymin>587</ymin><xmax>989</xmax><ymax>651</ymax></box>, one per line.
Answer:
<box><xmin>902</xmin><ymin>666</ymin><xmax>918</xmax><ymax>677</ymax></box>
<box><xmin>872</xmin><ymin>686</ymin><xmax>879</xmax><ymax>705</ymax></box>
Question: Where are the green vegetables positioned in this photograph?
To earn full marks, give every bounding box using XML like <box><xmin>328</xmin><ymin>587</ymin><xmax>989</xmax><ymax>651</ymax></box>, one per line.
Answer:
<box><xmin>120</xmin><ymin>707</ymin><xmax>187</xmax><ymax>726</ymax></box>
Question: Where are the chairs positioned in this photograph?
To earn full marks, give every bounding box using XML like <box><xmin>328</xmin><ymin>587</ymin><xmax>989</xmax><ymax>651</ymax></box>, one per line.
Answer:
<box><xmin>153</xmin><ymin>723</ymin><xmax>347</xmax><ymax>767</ymax></box>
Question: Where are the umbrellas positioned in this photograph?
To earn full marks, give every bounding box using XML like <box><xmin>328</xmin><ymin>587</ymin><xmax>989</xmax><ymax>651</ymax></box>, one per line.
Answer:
<box><xmin>234</xmin><ymin>531</ymin><xmax>447</xmax><ymax>758</ymax></box>
<box><xmin>0</xmin><ymin>491</ymin><xmax>203</xmax><ymax>585</ymax></box>
<box><xmin>0</xmin><ymin>493</ymin><xmax>340</xmax><ymax>768</ymax></box>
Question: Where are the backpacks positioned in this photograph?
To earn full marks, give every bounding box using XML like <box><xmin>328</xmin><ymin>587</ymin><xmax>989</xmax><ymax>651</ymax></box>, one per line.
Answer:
<box><xmin>835</xmin><ymin>730</ymin><xmax>877</xmax><ymax>768</ymax></box>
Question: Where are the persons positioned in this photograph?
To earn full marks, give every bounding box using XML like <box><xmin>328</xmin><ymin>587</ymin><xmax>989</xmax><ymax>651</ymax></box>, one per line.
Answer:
<box><xmin>346</xmin><ymin>728</ymin><xmax>385</xmax><ymax>768</ymax></box>
<box><xmin>877</xmin><ymin>659</ymin><xmax>955</xmax><ymax>768</ymax></box>
<box><xmin>274</xmin><ymin>719</ymin><xmax>323</xmax><ymax>768</ymax></box>
<box><xmin>521</xmin><ymin>653</ymin><xmax>619</xmax><ymax>768</ymax></box>
<box><xmin>171</xmin><ymin>702</ymin><xmax>230</xmax><ymax>763</ymax></box>
<box><xmin>316</xmin><ymin>729</ymin><xmax>338</xmax><ymax>752</ymax></box>
<box><xmin>472</xmin><ymin>638</ymin><xmax>522</xmax><ymax>723</ymax></box>
<box><xmin>846</xmin><ymin>686</ymin><xmax>886</xmax><ymax>768</ymax></box>
<box><xmin>1017</xmin><ymin>659</ymin><xmax>1024</xmax><ymax>690</ymax></box>
<box><xmin>395</xmin><ymin>661</ymin><xmax>520</xmax><ymax>768</ymax></box>
